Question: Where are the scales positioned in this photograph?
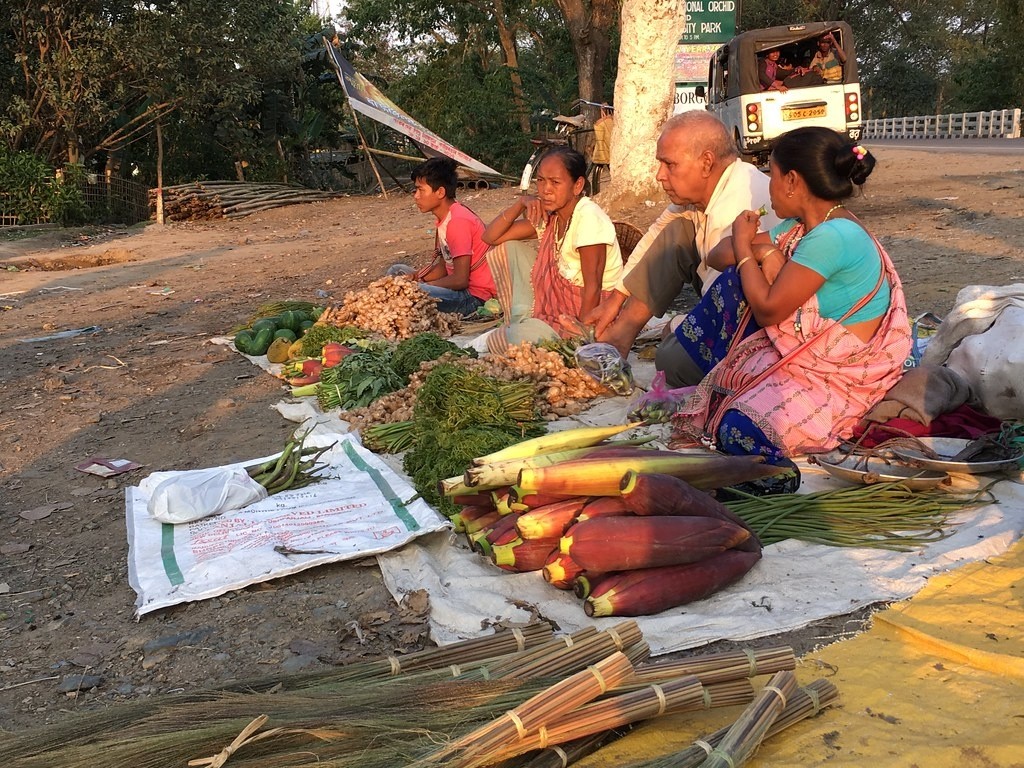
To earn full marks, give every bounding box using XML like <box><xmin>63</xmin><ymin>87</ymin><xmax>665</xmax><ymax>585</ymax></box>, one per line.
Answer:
<box><xmin>809</xmin><ymin>418</ymin><xmax>1024</xmax><ymax>492</ymax></box>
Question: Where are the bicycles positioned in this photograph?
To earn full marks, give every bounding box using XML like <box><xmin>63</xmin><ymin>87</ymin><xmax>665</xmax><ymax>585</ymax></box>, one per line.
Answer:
<box><xmin>519</xmin><ymin>98</ymin><xmax>616</xmax><ymax>219</ymax></box>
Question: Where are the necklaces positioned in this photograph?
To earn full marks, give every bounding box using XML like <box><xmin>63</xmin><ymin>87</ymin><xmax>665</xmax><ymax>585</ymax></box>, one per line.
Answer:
<box><xmin>787</xmin><ymin>202</ymin><xmax>848</xmax><ymax>258</ymax></box>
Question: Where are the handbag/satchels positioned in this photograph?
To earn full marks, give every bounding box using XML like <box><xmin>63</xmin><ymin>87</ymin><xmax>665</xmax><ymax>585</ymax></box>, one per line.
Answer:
<box><xmin>592</xmin><ymin>103</ymin><xmax>613</xmax><ymax>164</ymax></box>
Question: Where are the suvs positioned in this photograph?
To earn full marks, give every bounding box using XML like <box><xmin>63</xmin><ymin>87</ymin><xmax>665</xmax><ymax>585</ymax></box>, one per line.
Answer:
<box><xmin>694</xmin><ymin>20</ymin><xmax>865</xmax><ymax>172</ymax></box>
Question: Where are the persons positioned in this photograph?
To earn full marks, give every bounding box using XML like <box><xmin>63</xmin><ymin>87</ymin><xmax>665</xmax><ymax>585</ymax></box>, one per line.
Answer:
<box><xmin>758</xmin><ymin>48</ymin><xmax>806</xmax><ymax>93</ymax></box>
<box><xmin>667</xmin><ymin>123</ymin><xmax>914</xmax><ymax>468</ymax></box>
<box><xmin>381</xmin><ymin>154</ymin><xmax>503</xmax><ymax>316</ymax></box>
<box><xmin>795</xmin><ymin>29</ymin><xmax>849</xmax><ymax>84</ymax></box>
<box><xmin>461</xmin><ymin>147</ymin><xmax>625</xmax><ymax>356</ymax></box>
<box><xmin>556</xmin><ymin>107</ymin><xmax>795</xmax><ymax>386</ymax></box>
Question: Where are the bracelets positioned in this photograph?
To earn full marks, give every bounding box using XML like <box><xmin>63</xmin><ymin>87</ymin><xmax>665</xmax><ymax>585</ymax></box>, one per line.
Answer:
<box><xmin>756</xmin><ymin>247</ymin><xmax>779</xmax><ymax>265</ymax></box>
<box><xmin>500</xmin><ymin>210</ymin><xmax>514</xmax><ymax>224</ymax></box>
<box><xmin>734</xmin><ymin>256</ymin><xmax>755</xmax><ymax>277</ymax></box>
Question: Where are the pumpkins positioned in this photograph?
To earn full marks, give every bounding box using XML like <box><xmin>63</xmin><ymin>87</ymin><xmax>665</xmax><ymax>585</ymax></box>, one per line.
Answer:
<box><xmin>234</xmin><ymin>307</ymin><xmax>325</xmax><ymax>356</ymax></box>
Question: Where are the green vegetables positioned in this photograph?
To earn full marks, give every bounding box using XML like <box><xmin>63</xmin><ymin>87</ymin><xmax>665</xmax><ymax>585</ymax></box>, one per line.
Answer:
<box><xmin>362</xmin><ymin>362</ymin><xmax>549</xmax><ymax>516</ymax></box>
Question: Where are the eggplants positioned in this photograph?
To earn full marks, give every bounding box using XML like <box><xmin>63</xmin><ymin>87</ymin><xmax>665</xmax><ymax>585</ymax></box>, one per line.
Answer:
<box><xmin>244</xmin><ymin>418</ymin><xmax>340</xmax><ymax>497</ymax></box>
